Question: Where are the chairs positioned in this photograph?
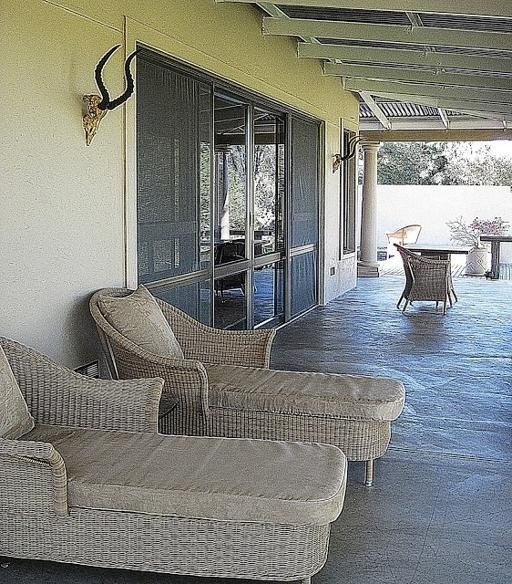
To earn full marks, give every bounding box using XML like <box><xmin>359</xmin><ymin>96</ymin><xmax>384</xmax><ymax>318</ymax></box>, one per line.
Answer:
<box><xmin>85</xmin><ymin>283</ymin><xmax>411</xmax><ymax>488</ymax></box>
<box><xmin>385</xmin><ymin>223</ymin><xmax>424</xmax><ymax>253</ymax></box>
<box><xmin>197</xmin><ymin>239</ymin><xmax>249</xmax><ymax>297</ymax></box>
<box><xmin>391</xmin><ymin>242</ymin><xmax>453</xmax><ymax>317</ymax></box>
<box><xmin>0</xmin><ymin>330</ymin><xmax>358</xmax><ymax>583</ymax></box>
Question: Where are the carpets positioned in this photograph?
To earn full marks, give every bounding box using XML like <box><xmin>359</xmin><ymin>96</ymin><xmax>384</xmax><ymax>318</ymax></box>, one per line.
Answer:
<box><xmin>212</xmin><ymin>298</ymin><xmax>249</xmax><ymax>330</ymax></box>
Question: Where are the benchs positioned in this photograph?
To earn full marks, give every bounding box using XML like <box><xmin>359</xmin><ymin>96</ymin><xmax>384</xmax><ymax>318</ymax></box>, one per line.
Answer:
<box><xmin>477</xmin><ymin>232</ymin><xmax>511</xmax><ymax>279</ymax></box>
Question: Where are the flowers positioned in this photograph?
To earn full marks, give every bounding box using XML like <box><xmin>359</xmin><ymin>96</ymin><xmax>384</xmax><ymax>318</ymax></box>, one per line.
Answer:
<box><xmin>444</xmin><ymin>211</ymin><xmax>511</xmax><ymax>249</ymax></box>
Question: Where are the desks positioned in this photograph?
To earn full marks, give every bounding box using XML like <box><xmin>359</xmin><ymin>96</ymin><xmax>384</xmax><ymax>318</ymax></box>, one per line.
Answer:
<box><xmin>400</xmin><ymin>242</ymin><xmax>474</xmax><ymax>307</ymax></box>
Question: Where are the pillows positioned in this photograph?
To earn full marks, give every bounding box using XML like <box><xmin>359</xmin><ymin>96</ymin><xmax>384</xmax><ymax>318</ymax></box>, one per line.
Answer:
<box><xmin>0</xmin><ymin>344</ymin><xmax>41</xmax><ymax>439</ymax></box>
<box><xmin>94</xmin><ymin>283</ymin><xmax>188</xmax><ymax>365</ymax></box>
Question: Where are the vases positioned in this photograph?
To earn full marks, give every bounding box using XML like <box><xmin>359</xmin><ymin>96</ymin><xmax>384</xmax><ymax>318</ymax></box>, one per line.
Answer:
<box><xmin>463</xmin><ymin>245</ymin><xmax>492</xmax><ymax>276</ymax></box>
